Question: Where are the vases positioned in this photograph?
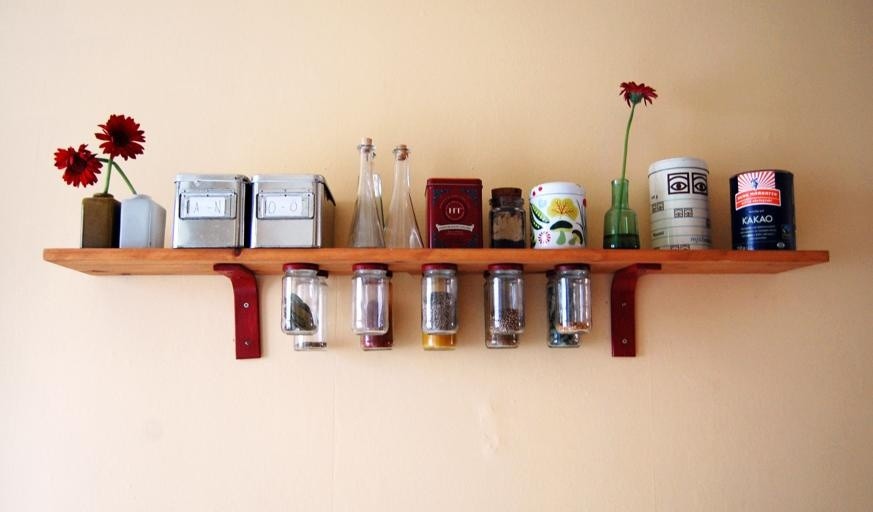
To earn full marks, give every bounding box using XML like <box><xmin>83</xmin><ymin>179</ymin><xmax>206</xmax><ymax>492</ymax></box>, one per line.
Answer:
<box><xmin>604</xmin><ymin>178</ymin><xmax>641</xmax><ymax>249</ymax></box>
<box><xmin>120</xmin><ymin>194</ymin><xmax>166</xmax><ymax>248</ymax></box>
<box><xmin>80</xmin><ymin>193</ymin><xmax>122</xmax><ymax>248</ymax></box>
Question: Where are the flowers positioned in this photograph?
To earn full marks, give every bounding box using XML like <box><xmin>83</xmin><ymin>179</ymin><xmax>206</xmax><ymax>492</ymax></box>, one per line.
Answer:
<box><xmin>54</xmin><ymin>143</ymin><xmax>137</xmax><ymax>196</ymax></box>
<box><xmin>95</xmin><ymin>114</ymin><xmax>146</xmax><ymax>192</ymax></box>
<box><xmin>612</xmin><ymin>81</ymin><xmax>659</xmax><ymax>245</ymax></box>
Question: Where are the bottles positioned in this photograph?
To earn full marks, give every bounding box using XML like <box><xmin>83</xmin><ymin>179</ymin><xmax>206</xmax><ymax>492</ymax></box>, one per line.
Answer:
<box><xmin>553</xmin><ymin>265</ymin><xmax>593</xmax><ymax>334</ymax></box>
<box><xmin>544</xmin><ymin>272</ymin><xmax>582</xmax><ymax>349</ymax></box>
<box><xmin>292</xmin><ymin>269</ymin><xmax>329</xmax><ymax>352</ymax></box>
<box><xmin>482</xmin><ymin>270</ymin><xmax>519</xmax><ymax>349</ymax></box>
<box><xmin>281</xmin><ymin>263</ymin><xmax>320</xmax><ymax>335</ymax></box>
<box><xmin>489</xmin><ymin>265</ymin><xmax>527</xmax><ymax>334</ymax></box>
<box><xmin>358</xmin><ymin>271</ymin><xmax>393</xmax><ymax>351</ymax></box>
<box><xmin>420</xmin><ymin>266</ymin><xmax>460</xmax><ymax>337</ymax></box>
<box><xmin>486</xmin><ymin>187</ymin><xmax>525</xmax><ymax>249</ymax></box>
<box><xmin>350</xmin><ymin>263</ymin><xmax>390</xmax><ymax>334</ymax></box>
<box><xmin>419</xmin><ymin>318</ymin><xmax>458</xmax><ymax>350</ymax></box>
<box><xmin>350</xmin><ymin>136</ymin><xmax>386</xmax><ymax>249</ymax></box>
<box><xmin>384</xmin><ymin>144</ymin><xmax>423</xmax><ymax>248</ymax></box>
